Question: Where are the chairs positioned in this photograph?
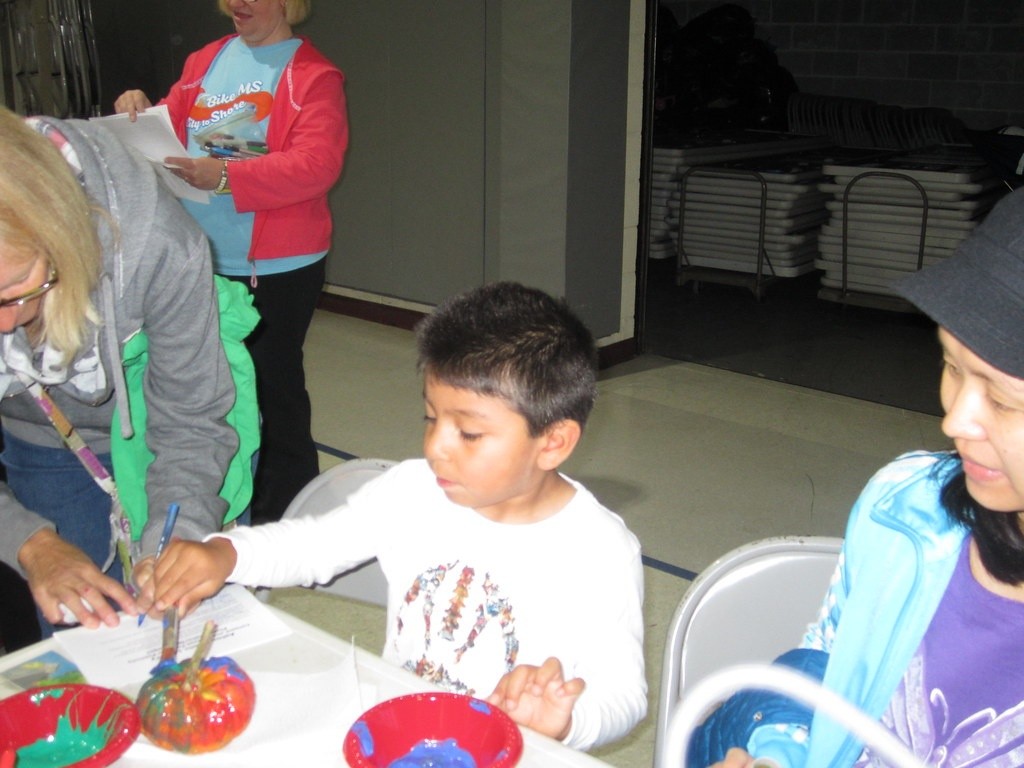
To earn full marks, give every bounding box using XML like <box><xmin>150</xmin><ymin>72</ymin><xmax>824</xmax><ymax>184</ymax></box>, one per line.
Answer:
<box><xmin>253</xmin><ymin>459</ymin><xmax>407</xmax><ymax>610</ymax></box>
<box><xmin>650</xmin><ymin>535</ymin><xmax>848</xmax><ymax>767</ymax></box>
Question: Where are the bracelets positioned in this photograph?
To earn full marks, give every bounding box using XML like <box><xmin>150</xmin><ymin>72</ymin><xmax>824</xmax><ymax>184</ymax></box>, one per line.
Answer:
<box><xmin>216</xmin><ymin>160</ymin><xmax>228</xmax><ymax>191</ymax></box>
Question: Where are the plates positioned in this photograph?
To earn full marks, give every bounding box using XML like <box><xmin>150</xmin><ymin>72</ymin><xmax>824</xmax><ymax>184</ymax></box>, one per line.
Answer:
<box><xmin>342</xmin><ymin>693</ymin><xmax>524</xmax><ymax>768</ymax></box>
<box><xmin>0</xmin><ymin>684</ymin><xmax>141</xmax><ymax>768</ymax></box>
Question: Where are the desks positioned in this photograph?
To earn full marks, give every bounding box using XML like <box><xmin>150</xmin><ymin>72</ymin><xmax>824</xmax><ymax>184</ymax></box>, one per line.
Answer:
<box><xmin>0</xmin><ymin>578</ymin><xmax>612</xmax><ymax>768</ymax></box>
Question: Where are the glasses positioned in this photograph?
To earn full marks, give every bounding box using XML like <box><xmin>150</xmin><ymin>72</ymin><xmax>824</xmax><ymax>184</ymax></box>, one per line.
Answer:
<box><xmin>0</xmin><ymin>269</ymin><xmax>58</xmax><ymax>306</ymax></box>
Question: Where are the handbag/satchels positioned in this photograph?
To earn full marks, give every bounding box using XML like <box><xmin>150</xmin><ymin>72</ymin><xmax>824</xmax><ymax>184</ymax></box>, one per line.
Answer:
<box><xmin>109</xmin><ymin>274</ymin><xmax>263</xmax><ymax>541</ymax></box>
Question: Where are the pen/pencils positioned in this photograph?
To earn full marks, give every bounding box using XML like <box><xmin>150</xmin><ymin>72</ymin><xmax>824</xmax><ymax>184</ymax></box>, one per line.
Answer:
<box><xmin>136</xmin><ymin>504</ymin><xmax>179</xmax><ymax>627</ymax></box>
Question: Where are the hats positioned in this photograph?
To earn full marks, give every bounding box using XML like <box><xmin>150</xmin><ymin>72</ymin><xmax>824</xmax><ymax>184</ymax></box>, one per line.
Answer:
<box><xmin>888</xmin><ymin>185</ymin><xmax>1024</xmax><ymax>379</ymax></box>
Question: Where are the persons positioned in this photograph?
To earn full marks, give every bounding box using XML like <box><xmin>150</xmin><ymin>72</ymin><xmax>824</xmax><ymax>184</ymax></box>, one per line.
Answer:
<box><xmin>154</xmin><ymin>281</ymin><xmax>647</xmax><ymax>752</ymax></box>
<box><xmin>709</xmin><ymin>182</ymin><xmax>1024</xmax><ymax>768</ymax></box>
<box><xmin>114</xmin><ymin>0</ymin><xmax>348</xmax><ymax>527</ymax></box>
<box><xmin>0</xmin><ymin>104</ymin><xmax>260</xmax><ymax>641</ymax></box>
